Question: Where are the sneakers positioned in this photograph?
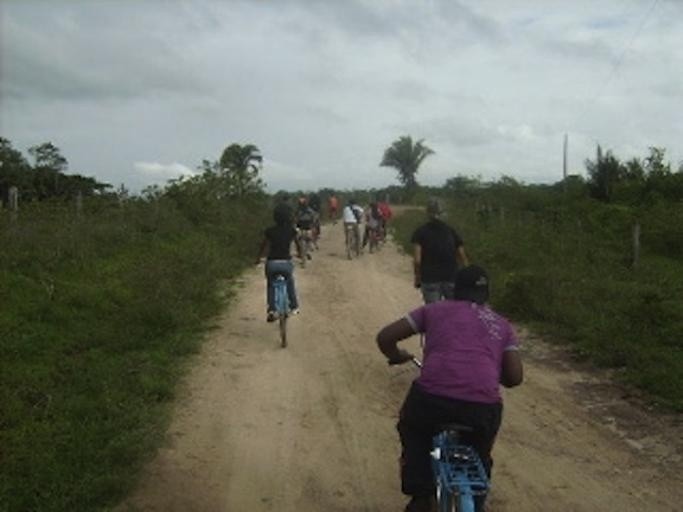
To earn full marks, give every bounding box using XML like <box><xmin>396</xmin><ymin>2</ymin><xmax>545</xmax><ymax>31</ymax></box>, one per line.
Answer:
<box><xmin>292</xmin><ymin>308</ymin><xmax>299</xmax><ymax>315</ymax></box>
<box><xmin>267</xmin><ymin>311</ymin><xmax>275</xmax><ymax>321</ymax></box>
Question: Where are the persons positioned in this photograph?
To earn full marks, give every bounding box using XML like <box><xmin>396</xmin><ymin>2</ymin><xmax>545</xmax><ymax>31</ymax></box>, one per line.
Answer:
<box><xmin>307</xmin><ymin>191</ymin><xmax>322</xmax><ymax>236</ymax></box>
<box><xmin>253</xmin><ymin>203</ymin><xmax>304</xmax><ymax>324</ymax></box>
<box><xmin>375</xmin><ymin>194</ymin><xmax>392</xmax><ymax>244</ymax></box>
<box><xmin>341</xmin><ymin>197</ymin><xmax>362</xmax><ymax>256</ymax></box>
<box><xmin>409</xmin><ymin>195</ymin><xmax>472</xmax><ymax>308</ymax></box>
<box><xmin>327</xmin><ymin>191</ymin><xmax>339</xmax><ymax>225</ymax></box>
<box><xmin>374</xmin><ymin>265</ymin><xmax>523</xmax><ymax>511</ymax></box>
<box><xmin>294</xmin><ymin>196</ymin><xmax>312</xmax><ymax>261</ymax></box>
<box><xmin>362</xmin><ymin>199</ymin><xmax>384</xmax><ymax>249</ymax></box>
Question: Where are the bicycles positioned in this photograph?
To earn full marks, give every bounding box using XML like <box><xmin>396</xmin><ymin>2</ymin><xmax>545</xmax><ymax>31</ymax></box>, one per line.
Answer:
<box><xmin>413</xmin><ymin>282</ymin><xmax>450</xmax><ymax>352</ymax></box>
<box><xmin>256</xmin><ymin>252</ymin><xmax>310</xmax><ymax>349</ymax></box>
<box><xmin>382</xmin><ymin>346</ymin><xmax>494</xmax><ymax>511</ymax></box>
<box><xmin>289</xmin><ymin>203</ymin><xmax>390</xmax><ymax>270</ymax></box>
